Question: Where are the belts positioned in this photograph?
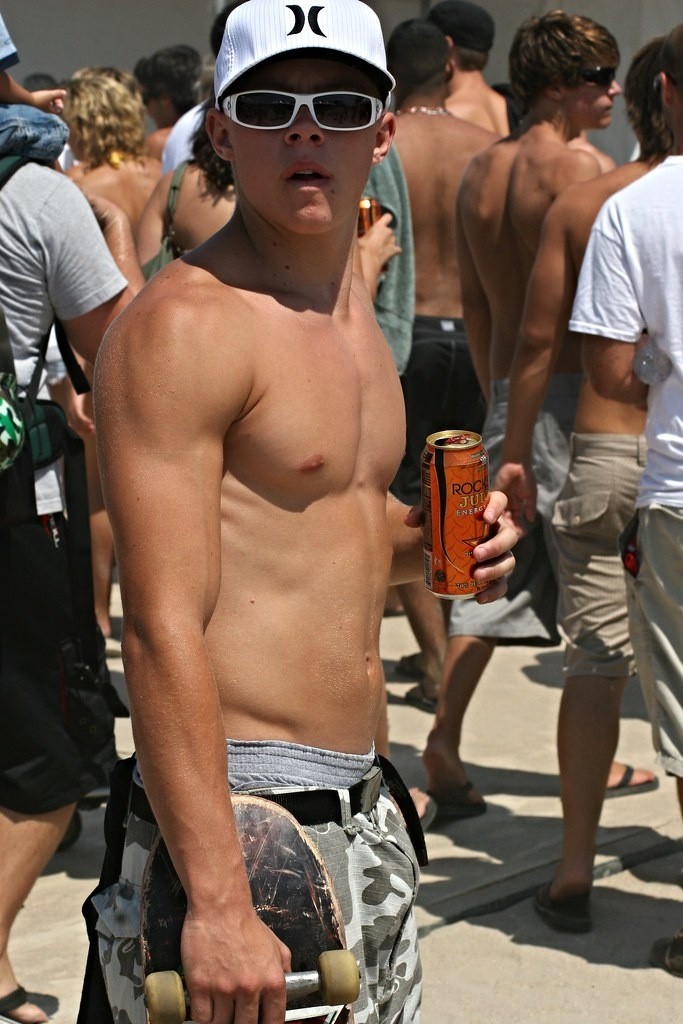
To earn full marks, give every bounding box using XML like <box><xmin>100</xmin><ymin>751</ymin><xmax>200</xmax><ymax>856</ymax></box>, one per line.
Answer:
<box><xmin>128</xmin><ymin>754</ymin><xmax>429</xmax><ymax>866</ymax></box>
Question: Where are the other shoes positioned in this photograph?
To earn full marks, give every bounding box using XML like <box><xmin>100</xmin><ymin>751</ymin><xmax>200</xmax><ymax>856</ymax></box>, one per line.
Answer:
<box><xmin>650</xmin><ymin>930</ymin><xmax>683</xmax><ymax>979</ymax></box>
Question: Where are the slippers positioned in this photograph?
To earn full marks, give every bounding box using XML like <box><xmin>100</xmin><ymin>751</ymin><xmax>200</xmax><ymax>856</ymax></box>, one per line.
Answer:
<box><xmin>404</xmin><ymin>682</ymin><xmax>437</xmax><ymax>713</ymax></box>
<box><xmin>401</xmin><ymin>788</ymin><xmax>437</xmax><ymax>836</ymax></box>
<box><xmin>395</xmin><ymin>655</ymin><xmax>425</xmax><ymax>679</ymax></box>
<box><xmin>533</xmin><ymin>880</ymin><xmax>592</xmax><ymax>934</ymax></box>
<box><xmin>434</xmin><ymin>780</ymin><xmax>487</xmax><ymax>817</ymax></box>
<box><xmin>603</xmin><ymin>765</ymin><xmax>659</xmax><ymax>798</ymax></box>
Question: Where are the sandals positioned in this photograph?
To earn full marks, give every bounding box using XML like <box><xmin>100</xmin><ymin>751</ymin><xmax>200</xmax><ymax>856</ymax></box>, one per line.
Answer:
<box><xmin>0</xmin><ymin>984</ymin><xmax>29</xmax><ymax>1024</ymax></box>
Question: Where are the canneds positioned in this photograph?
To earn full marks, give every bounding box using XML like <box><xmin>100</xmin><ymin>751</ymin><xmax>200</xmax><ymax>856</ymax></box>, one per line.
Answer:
<box><xmin>421</xmin><ymin>430</ymin><xmax>488</xmax><ymax>600</ymax></box>
<box><xmin>358</xmin><ymin>196</ymin><xmax>388</xmax><ymax>281</ymax></box>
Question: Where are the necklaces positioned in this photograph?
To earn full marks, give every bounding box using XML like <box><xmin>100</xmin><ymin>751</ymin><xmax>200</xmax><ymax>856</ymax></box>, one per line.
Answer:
<box><xmin>85</xmin><ymin>152</ymin><xmax>138</xmax><ymax>164</ymax></box>
<box><xmin>397</xmin><ymin>107</ymin><xmax>449</xmax><ymax>115</ymax></box>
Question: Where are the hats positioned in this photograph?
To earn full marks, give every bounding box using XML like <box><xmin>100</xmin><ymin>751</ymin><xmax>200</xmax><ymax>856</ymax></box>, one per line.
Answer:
<box><xmin>213</xmin><ymin>0</ymin><xmax>397</xmax><ymax>106</ymax></box>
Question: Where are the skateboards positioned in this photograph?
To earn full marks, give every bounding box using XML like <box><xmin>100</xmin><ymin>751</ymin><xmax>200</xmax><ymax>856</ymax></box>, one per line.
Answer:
<box><xmin>135</xmin><ymin>795</ymin><xmax>360</xmax><ymax>1024</ymax></box>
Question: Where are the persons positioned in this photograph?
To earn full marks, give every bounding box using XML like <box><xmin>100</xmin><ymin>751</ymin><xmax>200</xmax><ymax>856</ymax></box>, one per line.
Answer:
<box><xmin>493</xmin><ymin>37</ymin><xmax>673</xmax><ymax>928</ymax></box>
<box><xmin>570</xmin><ymin>26</ymin><xmax>682</xmax><ymax>975</ymax></box>
<box><xmin>0</xmin><ymin>0</ymin><xmax>518</xmax><ymax>1024</ymax></box>
<box><xmin>425</xmin><ymin>14</ymin><xmax>658</xmax><ymax>813</ymax></box>
<box><xmin>76</xmin><ymin>1</ymin><xmax>524</xmax><ymax>1023</ymax></box>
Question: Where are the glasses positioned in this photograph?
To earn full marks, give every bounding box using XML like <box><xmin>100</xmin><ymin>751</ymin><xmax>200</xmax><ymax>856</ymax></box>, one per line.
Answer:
<box><xmin>576</xmin><ymin>64</ymin><xmax>616</xmax><ymax>89</ymax></box>
<box><xmin>221</xmin><ymin>90</ymin><xmax>384</xmax><ymax>131</ymax></box>
